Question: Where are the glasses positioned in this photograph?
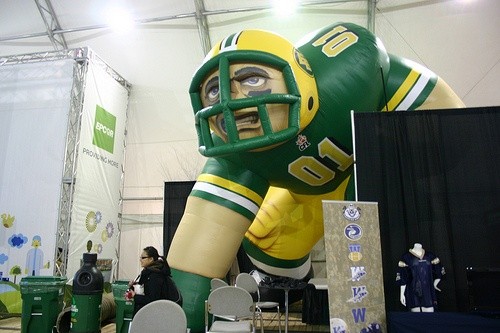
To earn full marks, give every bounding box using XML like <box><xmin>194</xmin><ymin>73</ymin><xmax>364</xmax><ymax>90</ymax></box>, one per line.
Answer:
<box><xmin>140</xmin><ymin>256</ymin><xmax>149</xmax><ymax>258</ymax></box>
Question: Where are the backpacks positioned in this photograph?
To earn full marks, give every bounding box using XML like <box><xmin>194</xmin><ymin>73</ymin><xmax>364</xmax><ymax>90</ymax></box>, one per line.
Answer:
<box><xmin>165</xmin><ymin>275</ymin><xmax>183</xmax><ymax>307</ymax></box>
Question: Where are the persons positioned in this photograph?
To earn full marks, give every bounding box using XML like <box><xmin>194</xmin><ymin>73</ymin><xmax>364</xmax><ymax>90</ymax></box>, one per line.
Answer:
<box><xmin>396</xmin><ymin>243</ymin><xmax>447</xmax><ymax>312</ymax></box>
<box><xmin>124</xmin><ymin>246</ymin><xmax>171</xmax><ymax>320</ymax></box>
<box><xmin>165</xmin><ymin>21</ymin><xmax>453</xmax><ymax>333</ymax></box>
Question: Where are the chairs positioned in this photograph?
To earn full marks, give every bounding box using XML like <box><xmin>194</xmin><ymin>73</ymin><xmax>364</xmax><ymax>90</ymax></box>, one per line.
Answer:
<box><xmin>204</xmin><ymin>273</ymin><xmax>282</xmax><ymax>333</ymax></box>
<box><xmin>128</xmin><ymin>300</ymin><xmax>190</xmax><ymax>333</ymax></box>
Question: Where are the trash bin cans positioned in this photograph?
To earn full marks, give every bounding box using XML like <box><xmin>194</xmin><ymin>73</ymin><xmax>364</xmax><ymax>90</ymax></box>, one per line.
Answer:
<box><xmin>114</xmin><ymin>298</ymin><xmax>135</xmax><ymax>333</ymax></box>
<box><xmin>19</xmin><ymin>276</ymin><xmax>67</xmax><ymax>333</ymax></box>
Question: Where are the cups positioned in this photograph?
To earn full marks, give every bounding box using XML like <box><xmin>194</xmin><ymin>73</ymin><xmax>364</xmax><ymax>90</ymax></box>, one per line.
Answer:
<box><xmin>125</xmin><ymin>289</ymin><xmax>132</xmax><ymax>305</ymax></box>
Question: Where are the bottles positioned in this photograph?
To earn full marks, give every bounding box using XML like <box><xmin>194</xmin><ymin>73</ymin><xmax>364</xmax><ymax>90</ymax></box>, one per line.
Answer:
<box><xmin>70</xmin><ymin>253</ymin><xmax>104</xmax><ymax>333</ymax></box>
